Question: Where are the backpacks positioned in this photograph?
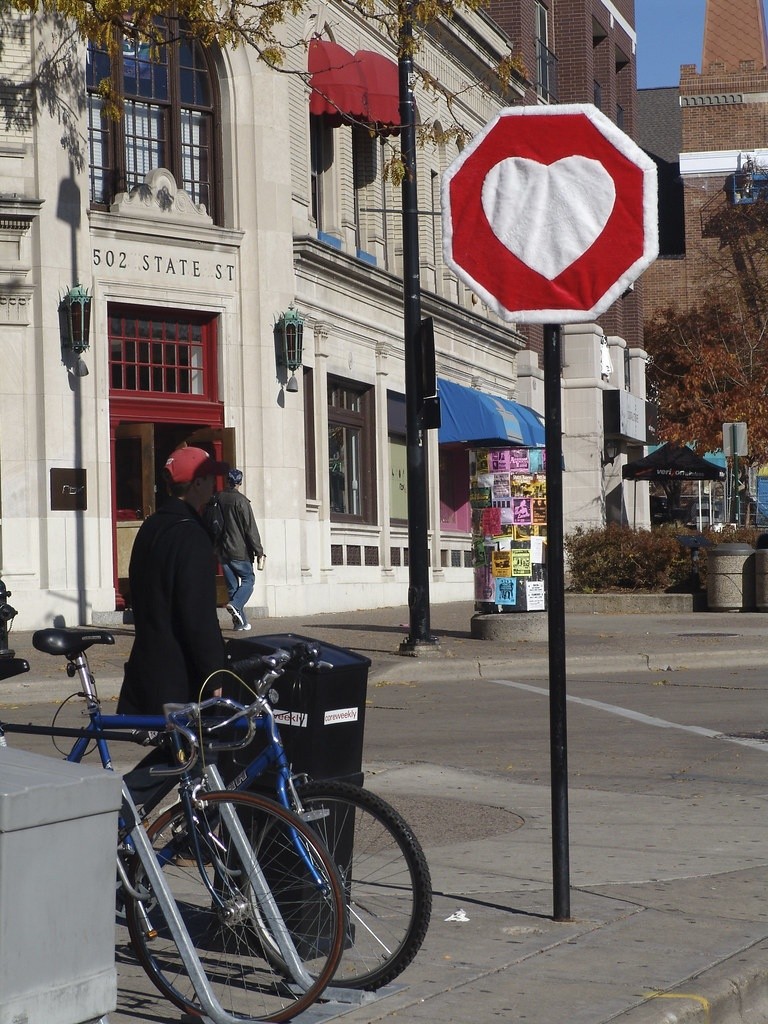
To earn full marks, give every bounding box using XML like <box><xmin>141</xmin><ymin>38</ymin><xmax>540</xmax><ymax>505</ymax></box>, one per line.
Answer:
<box><xmin>202</xmin><ymin>491</ymin><xmax>225</xmax><ymax>547</ymax></box>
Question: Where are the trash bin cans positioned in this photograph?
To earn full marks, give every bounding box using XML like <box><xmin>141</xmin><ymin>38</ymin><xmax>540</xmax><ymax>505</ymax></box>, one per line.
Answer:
<box><xmin>707</xmin><ymin>542</ymin><xmax>756</xmax><ymax>611</ymax></box>
<box><xmin>221</xmin><ymin>633</ymin><xmax>371</xmax><ymax>959</ymax></box>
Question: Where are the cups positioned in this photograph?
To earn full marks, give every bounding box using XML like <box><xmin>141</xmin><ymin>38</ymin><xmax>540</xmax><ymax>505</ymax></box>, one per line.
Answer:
<box><xmin>257</xmin><ymin>554</ymin><xmax>267</xmax><ymax>570</ymax></box>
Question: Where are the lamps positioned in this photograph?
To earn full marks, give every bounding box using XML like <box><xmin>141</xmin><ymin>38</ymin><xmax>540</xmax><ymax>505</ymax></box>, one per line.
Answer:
<box><xmin>600</xmin><ymin>441</ymin><xmax>618</xmax><ymax>468</ymax></box>
<box><xmin>279</xmin><ymin>300</ymin><xmax>311</xmax><ymax>393</ymax></box>
<box><xmin>63</xmin><ymin>284</ymin><xmax>95</xmax><ymax>376</ymax></box>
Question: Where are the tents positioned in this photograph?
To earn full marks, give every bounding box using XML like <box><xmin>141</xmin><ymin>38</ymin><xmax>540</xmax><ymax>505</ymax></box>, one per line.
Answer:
<box><xmin>620</xmin><ymin>440</ymin><xmax>727</xmax><ymax>530</ymax></box>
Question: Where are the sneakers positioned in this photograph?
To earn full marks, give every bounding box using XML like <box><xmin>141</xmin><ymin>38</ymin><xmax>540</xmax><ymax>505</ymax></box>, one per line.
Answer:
<box><xmin>226</xmin><ymin>603</ymin><xmax>244</xmax><ymax>626</ymax></box>
<box><xmin>234</xmin><ymin>623</ymin><xmax>251</xmax><ymax>631</ymax></box>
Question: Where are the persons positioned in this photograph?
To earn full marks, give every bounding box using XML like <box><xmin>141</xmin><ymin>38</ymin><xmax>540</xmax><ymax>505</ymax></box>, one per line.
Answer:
<box><xmin>117</xmin><ymin>446</ymin><xmax>229</xmax><ymax>867</ymax></box>
<box><xmin>515</xmin><ymin>500</ymin><xmax>529</xmax><ymax>519</ymax></box>
<box><xmin>201</xmin><ymin>469</ymin><xmax>266</xmax><ymax>633</ymax></box>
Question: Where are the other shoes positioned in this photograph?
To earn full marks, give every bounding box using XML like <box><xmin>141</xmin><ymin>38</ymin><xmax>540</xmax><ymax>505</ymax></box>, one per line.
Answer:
<box><xmin>176</xmin><ymin>848</ymin><xmax>216</xmax><ymax>868</ymax></box>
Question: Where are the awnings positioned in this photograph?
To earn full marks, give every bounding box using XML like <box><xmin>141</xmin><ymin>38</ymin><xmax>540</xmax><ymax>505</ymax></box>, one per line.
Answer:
<box><xmin>354</xmin><ymin>49</ymin><xmax>421</xmax><ymax>137</ymax></box>
<box><xmin>436</xmin><ymin>378</ymin><xmax>546</xmax><ymax>447</ymax></box>
<box><xmin>308</xmin><ymin>39</ymin><xmax>367</xmax><ymax>126</ymax></box>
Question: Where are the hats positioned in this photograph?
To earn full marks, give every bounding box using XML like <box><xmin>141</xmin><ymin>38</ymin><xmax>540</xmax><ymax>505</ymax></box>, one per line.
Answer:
<box><xmin>227</xmin><ymin>469</ymin><xmax>243</xmax><ymax>485</ymax></box>
<box><xmin>165</xmin><ymin>447</ymin><xmax>230</xmax><ymax>489</ymax></box>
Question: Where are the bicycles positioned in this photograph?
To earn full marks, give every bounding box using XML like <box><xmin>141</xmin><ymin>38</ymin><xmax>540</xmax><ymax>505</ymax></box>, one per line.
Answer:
<box><xmin>0</xmin><ymin>626</ymin><xmax>436</xmax><ymax>1024</ymax></box>
